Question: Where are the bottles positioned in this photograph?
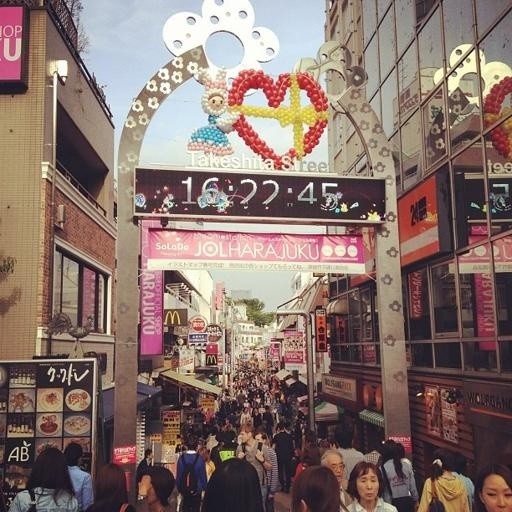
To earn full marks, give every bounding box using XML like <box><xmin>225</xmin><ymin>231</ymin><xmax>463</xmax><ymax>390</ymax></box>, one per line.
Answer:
<box><xmin>9</xmin><ymin>364</ymin><xmax>37</xmax><ymax>387</ymax></box>
<box><xmin>7</xmin><ymin>412</ymin><xmax>34</xmax><ymax>435</ymax></box>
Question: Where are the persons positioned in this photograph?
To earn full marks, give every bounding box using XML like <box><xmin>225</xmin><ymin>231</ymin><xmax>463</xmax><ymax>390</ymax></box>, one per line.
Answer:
<box><xmin>448</xmin><ymin>451</ymin><xmax>476</xmax><ymax>512</ymax></box>
<box><xmin>85</xmin><ymin>461</ymin><xmax>138</xmax><ymax>512</ymax></box>
<box><xmin>7</xmin><ymin>447</ymin><xmax>80</xmax><ymax>512</ymax></box>
<box><xmin>375</xmin><ymin>439</ymin><xmax>420</xmax><ymax>512</ymax></box>
<box><xmin>472</xmin><ymin>463</ymin><xmax>512</xmax><ymax>512</ymax></box>
<box><xmin>63</xmin><ymin>442</ymin><xmax>94</xmax><ymax>512</ymax></box>
<box><xmin>417</xmin><ymin>447</ymin><xmax>471</xmax><ymax>512</ymax></box>
<box><xmin>132</xmin><ymin>350</ymin><xmax>399</xmax><ymax>511</ymax></box>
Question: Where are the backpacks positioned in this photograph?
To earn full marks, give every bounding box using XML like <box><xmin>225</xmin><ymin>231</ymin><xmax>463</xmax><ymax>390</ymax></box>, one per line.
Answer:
<box><xmin>181</xmin><ymin>454</ymin><xmax>199</xmax><ymax>497</ymax></box>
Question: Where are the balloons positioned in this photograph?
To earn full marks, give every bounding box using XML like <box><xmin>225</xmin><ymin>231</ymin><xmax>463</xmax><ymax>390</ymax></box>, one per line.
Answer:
<box><xmin>482</xmin><ymin>74</ymin><xmax>512</xmax><ymax>165</ymax></box>
<box><xmin>186</xmin><ymin>64</ymin><xmax>329</xmax><ymax>170</ymax></box>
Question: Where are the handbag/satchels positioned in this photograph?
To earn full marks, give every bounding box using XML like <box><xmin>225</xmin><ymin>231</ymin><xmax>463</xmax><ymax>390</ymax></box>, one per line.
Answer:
<box><xmin>429</xmin><ymin>496</ymin><xmax>445</xmax><ymax>512</ymax></box>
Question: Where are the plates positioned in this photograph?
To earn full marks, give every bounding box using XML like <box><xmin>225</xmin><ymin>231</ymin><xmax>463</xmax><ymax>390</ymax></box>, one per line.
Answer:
<box><xmin>65</xmin><ymin>388</ymin><xmax>91</xmax><ymax>411</ymax></box>
<box><xmin>64</xmin><ymin>416</ymin><xmax>91</xmax><ymax>434</ymax></box>
<box><xmin>38</xmin><ymin>390</ymin><xmax>62</xmax><ymax>411</ymax></box>
<box><xmin>36</xmin><ymin>413</ymin><xmax>62</xmax><ymax>435</ymax></box>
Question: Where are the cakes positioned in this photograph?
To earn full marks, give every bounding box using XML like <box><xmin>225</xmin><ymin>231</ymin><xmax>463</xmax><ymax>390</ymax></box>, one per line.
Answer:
<box><xmin>41</xmin><ymin>390</ymin><xmax>61</xmax><ymax>410</ymax></box>
